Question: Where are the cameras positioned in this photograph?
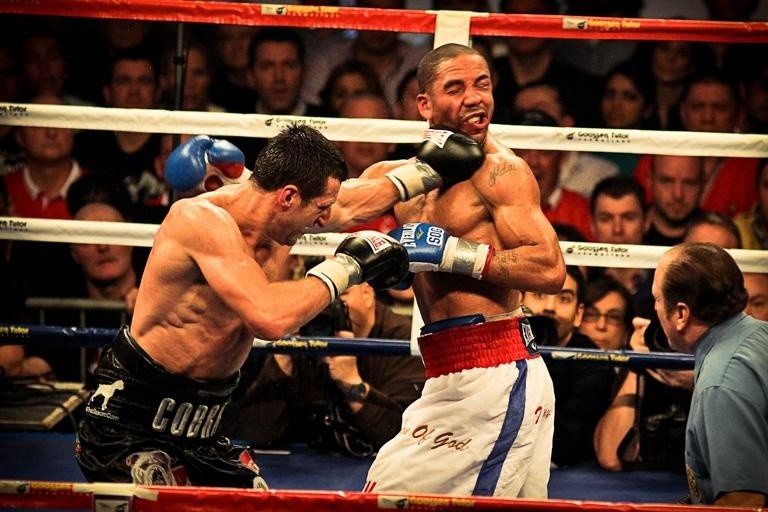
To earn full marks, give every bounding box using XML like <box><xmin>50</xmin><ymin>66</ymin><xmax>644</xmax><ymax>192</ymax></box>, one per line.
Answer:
<box><xmin>531</xmin><ymin>315</ymin><xmax>560</xmax><ymax>346</ymax></box>
<box><xmin>645</xmin><ymin>318</ymin><xmax>674</xmax><ymax>352</ymax></box>
<box><xmin>299</xmin><ymin>299</ymin><xmax>352</xmax><ymax>336</ymax></box>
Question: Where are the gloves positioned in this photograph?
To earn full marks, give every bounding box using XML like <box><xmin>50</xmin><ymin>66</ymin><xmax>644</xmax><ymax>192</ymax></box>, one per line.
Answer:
<box><xmin>164</xmin><ymin>135</ymin><xmax>254</xmax><ymax>194</ymax></box>
<box><xmin>385</xmin><ymin>126</ymin><xmax>486</xmax><ymax>203</ymax></box>
<box><xmin>384</xmin><ymin>223</ymin><xmax>495</xmax><ymax>283</ymax></box>
<box><xmin>306</xmin><ymin>229</ymin><xmax>410</xmax><ymax>305</ymax></box>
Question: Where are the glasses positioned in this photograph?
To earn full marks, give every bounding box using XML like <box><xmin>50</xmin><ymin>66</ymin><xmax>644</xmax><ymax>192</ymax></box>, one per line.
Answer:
<box><xmin>581</xmin><ymin>306</ymin><xmax>630</xmax><ymax>326</ymax></box>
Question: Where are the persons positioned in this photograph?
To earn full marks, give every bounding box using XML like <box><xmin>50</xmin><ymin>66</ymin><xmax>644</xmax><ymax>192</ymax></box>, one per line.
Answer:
<box><xmin>506</xmin><ymin>111</ymin><xmax>599</xmax><ymax>242</ymax></box>
<box><xmin>338</xmin><ymin>89</ymin><xmax>398</xmax><ymax>178</ymax></box>
<box><xmin>77</xmin><ymin>54</ymin><xmax>176</xmax><ymax>220</ymax></box>
<box><xmin>216</xmin><ymin>13</ymin><xmax>254</xmax><ymax>118</ymax></box>
<box><xmin>730</xmin><ymin>207</ymin><xmax>768</xmax><ymax>254</ymax></box>
<box><xmin>583</xmin><ymin>175</ymin><xmax>662</xmax><ymax>321</ymax></box>
<box><xmin>633</xmin><ymin>66</ymin><xmax>759</xmax><ymax>214</ymax></box>
<box><xmin>333</xmin><ymin>45</ymin><xmax>570</xmax><ymax>498</ymax></box>
<box><xmin>647</xmin><ymin>15</ymin><xmax>709</xmax><ymax>139</ymax></box>
<box><xmin>350</xmin><ymin>0</ymin><xmax>429</xmax><ymax>118</ymax></box>
<box><xmin>654</xmin><ymin>241</ymin><xmax>768</xmax><ymax>509</ymax></box>
<box><xmin>736</xmin><ymin>157</ymin><xmax>768</xmax><ymax>259</ymax></box>
<box><xmin>161</xmin><ymin>43</ymin><xmax>231</xmax><ymax>148</ymax></box>
<box><xmin>13</xmin><ymin>34</ymin><xmax>69</xmax><ymax>93</ymax></box>
<box><xmin>587</xmin><ymin>62</ymin><xmax>661</xmax><ymax>178</ymax></box>
<box><xmin>73</xmin><ymin>124</ymin><xmax>484</xmax><ymax>488</ymax></box>
<box><xmin>490</xmin><ymin>0</ymin><xmax>603</xmax><ymax>129</ymax></box>
<box><xmin>510</xmin><ymin>77</ymin><xmax>631</xmax><ymax>199</ymax></box>
<box><xmin>576</xmin><ymin>275</ymin><xmax>634</xmax><ymax>356</ymax></box>
<box><xmin>0</xmin><ymin>94</ymin><xmax>91</xmax><ymax>239</ymax></box>
<box><xmin>245</xmin><ymin>28</ymin><xmax>338</xmax><ymax>164</ymax></box>
<box><xmin>1</xmin><ymin>206</ymin><xmax>142</xmax><ymax>380</ymax></box>
<box><xmin>734</xmin><ymin>268</ymin><xmax>767</xmax><ymax>330</ymax></box>
<box><xmin>240</xmin><ymin>277</ymin><xmax>426</xmax><ymax>446</ymax></box>
<box><xmin>316</xmin><ymin>59</ymin><xmax>385</xmax><ymax>106</ymax></box>
<box><xmin>519</xmin><ymin>265</ymin><xmax>617</xmax><ymax>468</ymax></box>
<box><xmin>594</xmin><ymin>214</ymin><xmax>743</xmax><ymax>471</ymax></box>
<box><xmin>646</xmin><ymin>140</ymin><xmax>735</xmax><ymax>255</ymax></box>
<box><xmin>398</xmin><ymin>63</ymin><xmax>427</xmax><ymax>134</ymax></box>
